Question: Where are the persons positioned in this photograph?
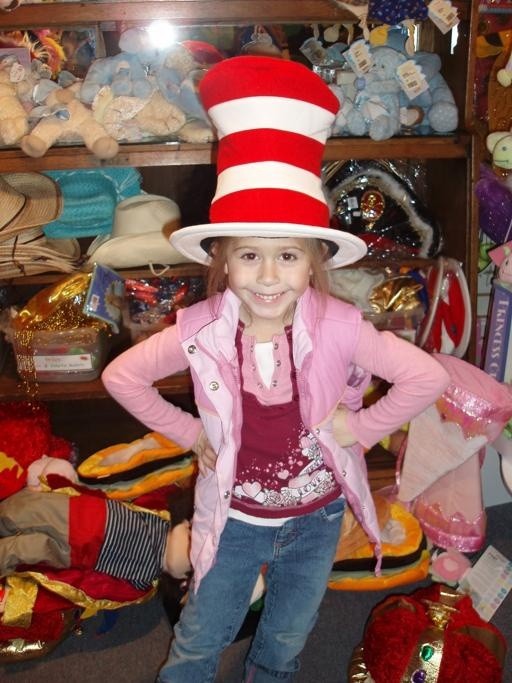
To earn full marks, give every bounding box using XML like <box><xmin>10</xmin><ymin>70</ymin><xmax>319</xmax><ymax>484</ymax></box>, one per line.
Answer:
<box><xmin>0</xmin><ymin>492</ymin><xmax>191</xmax><ymax>592</ymax></box>
<box><xmin>99</xmin><ymin>235</ymin><xmax>450</xmax><ymax>681</ymax></box>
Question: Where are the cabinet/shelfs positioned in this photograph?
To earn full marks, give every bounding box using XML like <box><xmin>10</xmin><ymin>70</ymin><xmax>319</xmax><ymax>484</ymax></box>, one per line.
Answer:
<box><xmin>0</xmin><ymin>3</ymin><xmax>511</xmax><ymax>481</ymax></box>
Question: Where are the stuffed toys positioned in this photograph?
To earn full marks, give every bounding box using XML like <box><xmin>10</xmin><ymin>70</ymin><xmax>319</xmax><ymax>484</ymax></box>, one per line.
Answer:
<box><xmin>0</xmin><ymin>21</ymin><xmax>457</xmax><ymax>159</ymax></box>
<box><xmin>474</xmin><ymin>49</ymin><xmax>512</xmax><ymax>292</ymax></box>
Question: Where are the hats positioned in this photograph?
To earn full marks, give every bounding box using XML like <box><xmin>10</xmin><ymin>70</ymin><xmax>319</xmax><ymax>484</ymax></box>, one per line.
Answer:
<box><xmin>170</xmin><ymin>53</ymin><xmax>369</xmax><ymax>269</ymax></box>
<box><xmin>0</xmin><ymin>168</ymin><xmax>196</xmax><ymax>274</ymax></box>
